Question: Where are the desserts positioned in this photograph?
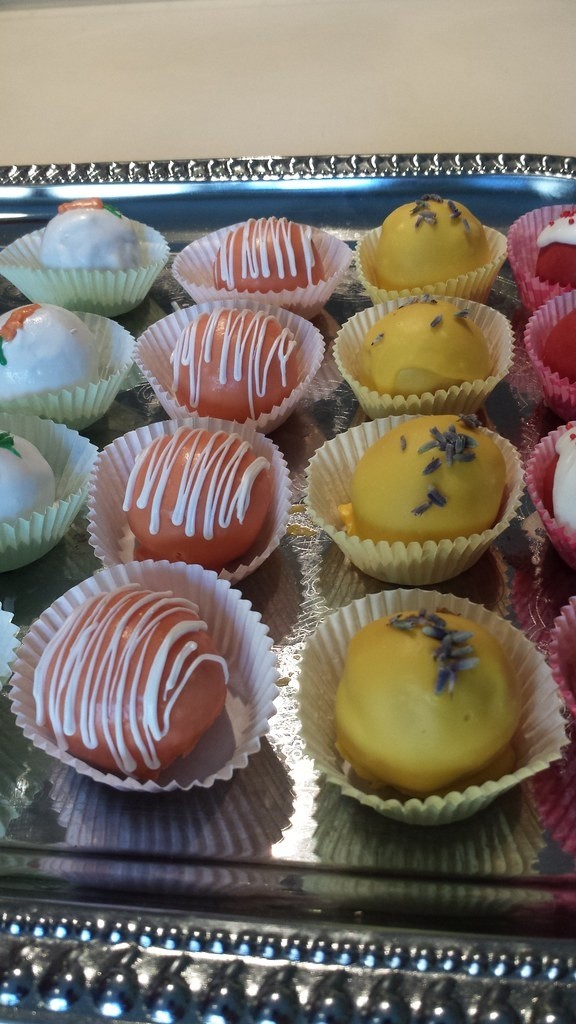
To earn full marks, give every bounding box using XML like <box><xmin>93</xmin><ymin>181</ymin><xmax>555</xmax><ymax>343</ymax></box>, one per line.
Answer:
<box><xmin>0</xmin><ymin>193</ymin><xmax>576</xmax><ymax>824</ymax></box>
